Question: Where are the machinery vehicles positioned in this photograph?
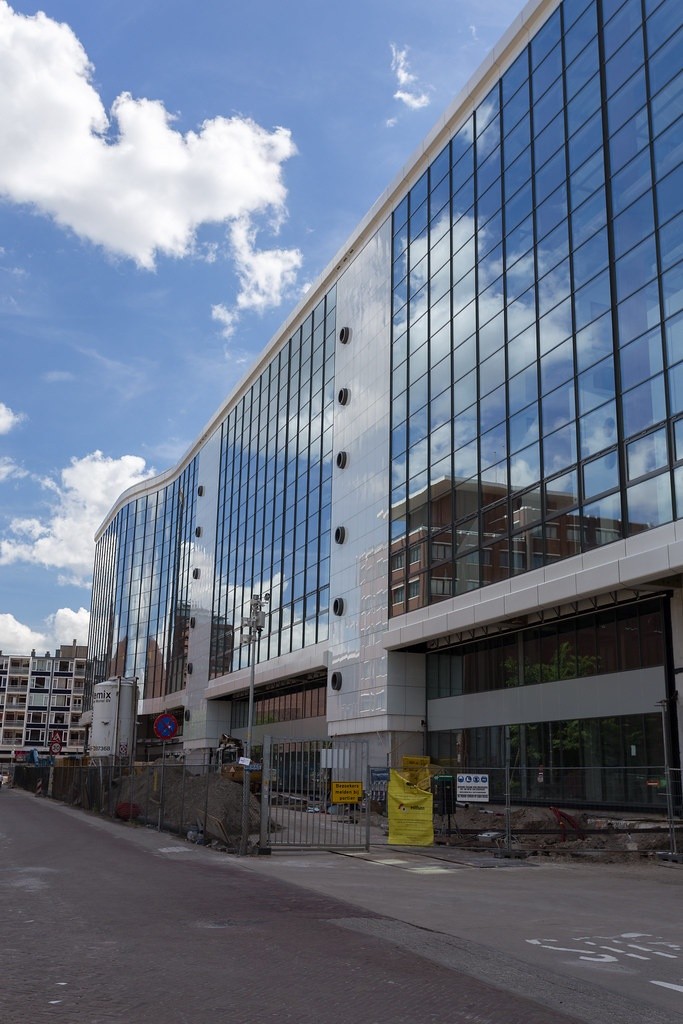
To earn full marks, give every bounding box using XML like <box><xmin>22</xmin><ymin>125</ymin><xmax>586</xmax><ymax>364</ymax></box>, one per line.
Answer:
<box><xmin>216</xmin><ymin>744</ymin><xmax>263</xmax><ymax>781</ymax></box>
